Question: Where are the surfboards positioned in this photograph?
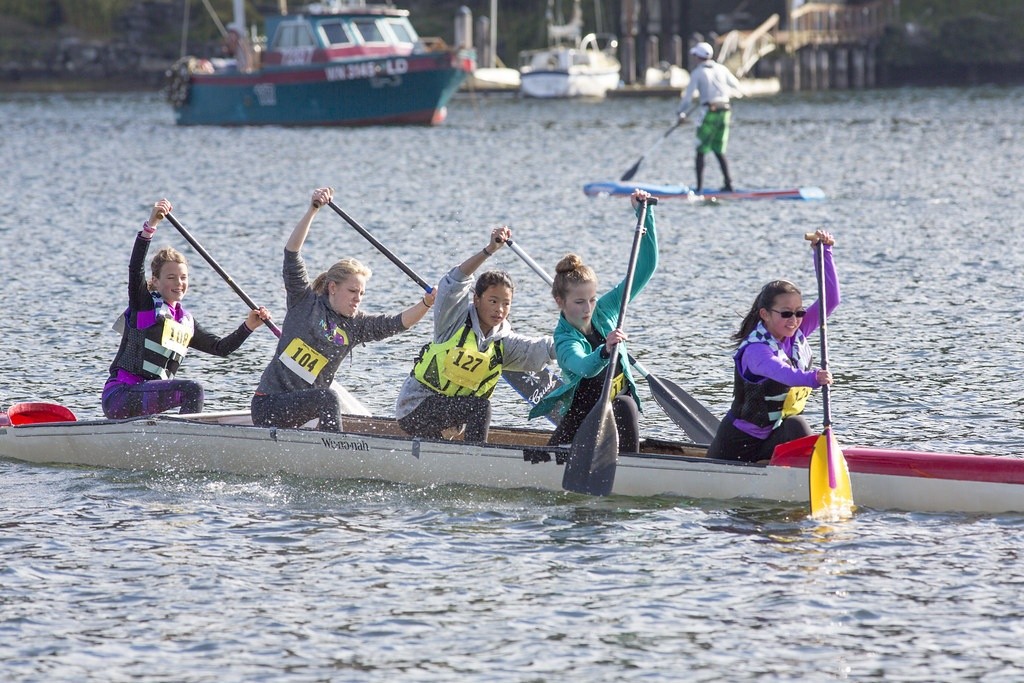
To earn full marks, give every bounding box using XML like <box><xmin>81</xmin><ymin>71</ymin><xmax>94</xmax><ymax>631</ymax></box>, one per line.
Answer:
<box><xmin>582</xmin><ymin>181</ymin><xmax>826</xmax><ymax>201</ymax></box>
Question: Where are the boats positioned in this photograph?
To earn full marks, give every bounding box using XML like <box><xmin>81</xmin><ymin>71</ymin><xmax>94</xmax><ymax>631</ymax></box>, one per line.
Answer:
<box><xmin>172</xmin><ymin>0</ymin><xmax>475</xmax><ymax>130</ymax></box>
<box><xmin>462</xmin><ymin>64</ymin><xmax>522</xmax><ymax>90</ymax></box>
<box><xmin>1</xmin><ymin>403</ymin><xmax>1024</xmax><ymax>516</ymax></box>
<box><xmin>736</xmin><ymin>76</ymin><xmax>781</xmax><ymax>100</ymax></box>
<box><xmin>520</xmin><ymin>21</ymin><xmax>622</xmax><ymax>100</ymax></box>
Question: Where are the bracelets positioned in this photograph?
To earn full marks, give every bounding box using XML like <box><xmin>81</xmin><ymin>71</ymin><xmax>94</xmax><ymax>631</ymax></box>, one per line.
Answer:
<box><xmin>423</xmin><ymin>298</ymin><xmax>431</xmax><ymax>307</ymax></box>
<box><xmin>143</xmin><ymin>221</ymin><xmax>156</xmax><ymax>232</ymax></box>
<box><xmin>600</xmin><ymin>346</ymin><xmax>611</xmax><ymax>359</ymax></box>
<box><xmin>484</xmin><ymin>248</ymin><xmax>492</xmax><ymax>255</ymax></box>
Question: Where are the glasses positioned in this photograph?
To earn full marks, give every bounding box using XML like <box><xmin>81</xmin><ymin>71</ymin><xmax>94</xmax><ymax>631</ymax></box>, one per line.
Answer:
<box><xmin>771</xmin><ymin>308</ymin><xmax>807</xmax><ymax>318</ymax></box>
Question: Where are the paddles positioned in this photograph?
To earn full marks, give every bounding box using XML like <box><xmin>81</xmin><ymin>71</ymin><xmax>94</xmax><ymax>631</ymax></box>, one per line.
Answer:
<box><xmin>312</xmin><ymin>194</ymin><xmax>564</xmax><ymax>426</ymax></box>
<box><xmin>156</xmin><ymin>207</ymin><xmax>373</xmax><ymax>418</ymax></box>
<box><xmin>621</xmin><ymin>103</ymin><xmax>700</xmax><ymax>181</ymax></box>
<box><xmin>494</xmin><ymin>231</ymin><xmax>723</xmax><ymax>444</ymax></box>
<box><xmin>808</xmin><ymin>239</ymin><xmax>855</xmax><ymax>520</ymax></box>
<box><xmin>560</xmin><ymin>194</ymin><xmax>660</xmax><ymax>496</ymax></box>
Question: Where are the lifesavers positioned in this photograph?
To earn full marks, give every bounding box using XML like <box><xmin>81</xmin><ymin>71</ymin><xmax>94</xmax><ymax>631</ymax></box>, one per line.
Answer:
<box><xmin>163</xmin><ymin>64</ymin><xmax>190</xmax><ymax>110</ymax></box>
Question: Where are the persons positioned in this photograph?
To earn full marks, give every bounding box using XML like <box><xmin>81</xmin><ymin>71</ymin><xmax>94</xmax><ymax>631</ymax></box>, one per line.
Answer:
<box><xmin>528</xmin><ymin>189</ymin><xmax>657</xmax><ymax>452</ymax></box>
<box><xmin>250</xmin><ymin>187</ymin><xmax>438</xmax><ymax>433</ymax></box>
<box><xmin>707</xmin><ymin>230</ymin><xmax>840</xmax><ymax>463</ymax></box>
<box><xmin>675</xmin><ymin>43</ymin><xmax>742</xmax><ymax>193</ymax></box>
<box><xmin>395</xmin><ymin>226</ymin><xmax>557</xmax><ymax>442</ymax></box>
<box><xmin>101</xmin><ymin>197</ymin><xmax>272</xmax><ymax>419</ymax></box>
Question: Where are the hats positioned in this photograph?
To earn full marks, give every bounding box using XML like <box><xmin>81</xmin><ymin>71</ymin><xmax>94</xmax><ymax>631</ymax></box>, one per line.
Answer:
<box><xmin>690</xmin><ymin>42</ymin><xmax>713</xmax><ymax>59</ymax></box>
